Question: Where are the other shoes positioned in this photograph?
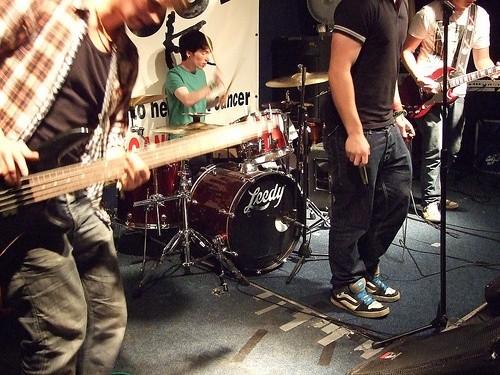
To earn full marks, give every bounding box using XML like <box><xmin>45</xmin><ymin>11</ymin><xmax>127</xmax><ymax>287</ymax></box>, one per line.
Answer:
<box><xmin>423</xmin><ymin>202</ymin><xmax>440</xmax><ymax>222</ymax></box>
<box><xmin>446</xmin><ymin>199</ymin><xmax>458</xmax><ymax>209</ymax></box>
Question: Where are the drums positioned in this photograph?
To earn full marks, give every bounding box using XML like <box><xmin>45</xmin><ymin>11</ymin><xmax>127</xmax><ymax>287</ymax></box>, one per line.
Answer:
<box><xmin>228</xmin><ymin>107</ymin><xmax>295</xmax><ymax>162</ymax></box>
<box><xmin>113</xmin><ymin>162</ymin><xmax>184</xmax><ymax>230</ymax></box>
<box><xmin>184</xmin><ymin>162</ymin><xmax>306</xmax><ymax>276</ymax></box>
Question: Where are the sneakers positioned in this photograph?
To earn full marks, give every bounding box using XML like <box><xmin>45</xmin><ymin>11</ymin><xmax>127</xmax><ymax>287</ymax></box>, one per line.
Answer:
<box><xmin>366</xmin><ymin>274</ymin><xmax>400</xmax><ymax>302</ymax></box>
<box><xmin>331</xmin><ymin>278</ymin><xmax>390</xmax><ymax>318</ymax></box>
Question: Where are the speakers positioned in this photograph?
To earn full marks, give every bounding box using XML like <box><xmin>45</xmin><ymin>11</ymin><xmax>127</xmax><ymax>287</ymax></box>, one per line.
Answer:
<box><xmin>348</xmin><ymin>316</ymin><xmax>500</xmax><ymax>375</ymax></box>
<box><xmin>308</xmin><ymin>141</ymin><xmax>333</xmax><ymax>211</ymax></box>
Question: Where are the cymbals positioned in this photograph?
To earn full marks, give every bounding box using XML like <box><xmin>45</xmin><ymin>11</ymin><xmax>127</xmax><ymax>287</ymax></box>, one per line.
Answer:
<box><xmin>261</xmin><ymin>100</ymin><xmax>314</xmax><ymax>109</ymax></box>
<box><xmin>265</xmin><ymin>70</ymin><xmax>328</xmax><ymax>87</ymax></box>
<box><xmin>131</xmin><ymin>93</ymin><xmax>166</xmax><ymax>106</ymax></box>
<box><xmin>152</xmin><ymin>121</ymin><xmax>227</xmax><ymax>135</ymax></box>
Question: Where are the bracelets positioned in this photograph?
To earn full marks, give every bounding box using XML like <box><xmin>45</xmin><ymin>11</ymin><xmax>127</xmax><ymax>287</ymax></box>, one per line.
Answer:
<box><xmin>392</xmin><ymin>109</ymin><xmax>407</xmax><ymax>119</ymax></box>
<box><xmin>209</xmin><ymin>83</ymin><xmax>216</xmax><ymax>90</ymax></box>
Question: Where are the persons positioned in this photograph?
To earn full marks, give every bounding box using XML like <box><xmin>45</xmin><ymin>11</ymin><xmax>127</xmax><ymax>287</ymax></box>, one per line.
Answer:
<box><xmin>400</xmin><ymin>0</ymin><xmax>500</xmax><ymax>223</ymax></box>
<box><xmin>165</xmin><ymin>29</ymin><xmax>228</xmax><ymax>181</ymax></box>
<box><xmin>319</xmin><ymin>0</ymin><xmax>416</xmax><ymax>317</ymax></box>
<box><xmin>0</xmin><ymin>0</ymin><xmax>178</xmax><ymax>375</ymax></box>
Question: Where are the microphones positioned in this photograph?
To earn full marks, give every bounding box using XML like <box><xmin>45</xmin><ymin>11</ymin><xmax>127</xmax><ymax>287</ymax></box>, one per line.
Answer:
<box><xmin>283</xmin><ymin>216</ymin><xmax>310</xmax><ymax>230</ymax></box>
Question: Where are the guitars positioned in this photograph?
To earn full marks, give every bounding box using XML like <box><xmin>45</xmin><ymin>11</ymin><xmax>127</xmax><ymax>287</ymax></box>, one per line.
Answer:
<box><xmin>1</xmin><ymin>101</ymin><xmax>282</xmax><ymax>293</ymax></box>
<box><xmin>398</xmin><ymin>60</ymin><xmax>500</xmax><ymax>119</ymax></box>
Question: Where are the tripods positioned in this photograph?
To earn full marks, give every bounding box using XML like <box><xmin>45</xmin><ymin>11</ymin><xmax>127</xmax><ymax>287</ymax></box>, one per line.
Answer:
<box><xmin>133</xmin><ymin>158</ymin><xmax>250</xmax><ymax>300</ymax></box>
<box><xmin>284</xmin><ymin>66</ymin><xmax>333</xmax><ymax>284</ymax></box>
